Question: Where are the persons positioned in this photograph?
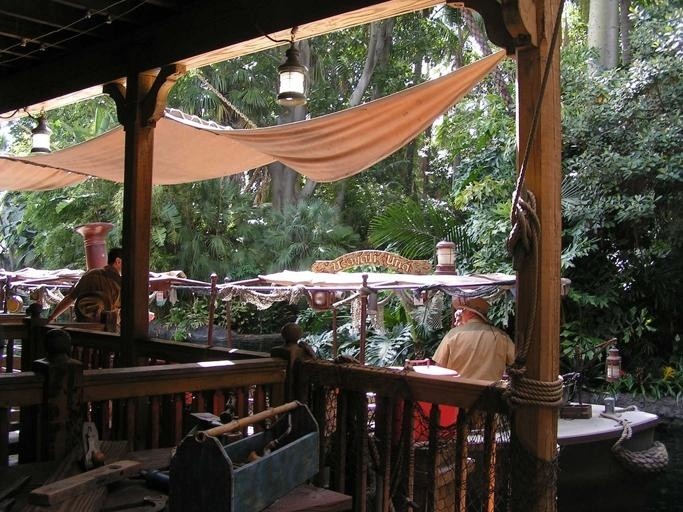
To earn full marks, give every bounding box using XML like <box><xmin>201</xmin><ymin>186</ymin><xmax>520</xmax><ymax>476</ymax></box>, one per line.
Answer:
<box><xmin>430</xmin><ymin>295</ymin><xmax>514</xmax><ymax>430</ymax></box>
<box><xmin>46</xmin><ymin>246</ymin><xmax>122</xmax><ymax>325</ymax></box>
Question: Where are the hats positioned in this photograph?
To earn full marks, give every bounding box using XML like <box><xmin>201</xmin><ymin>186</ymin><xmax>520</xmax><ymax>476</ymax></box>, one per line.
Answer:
<box><xmin>450</xmin><ymin>295</ymin><xmax>489</xmax><ymax>322</ymax></box>
<box><xmin>74</xmin><ymin>291</ymin><xmax>111</xmax><ymax>322</ymax></box>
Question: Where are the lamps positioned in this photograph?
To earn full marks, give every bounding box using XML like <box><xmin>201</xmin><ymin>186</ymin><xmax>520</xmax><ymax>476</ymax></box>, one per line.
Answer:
<box><xmin>21</xmin><ymin>106</ymin><xmax>55</xmax><ymax>155</ymax></box>
<box><xmin>264</xmin><ymin>27</ymin><xmax>308</xmax><ymax>108</ymax></box>
<box><xmin>604</xmin><ymin>342</ymin><xmax>622</xmax><ymax>383</ymax></box>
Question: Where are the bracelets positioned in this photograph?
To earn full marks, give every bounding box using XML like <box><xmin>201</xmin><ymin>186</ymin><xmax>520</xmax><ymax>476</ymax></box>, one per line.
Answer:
<box><xmin>47</xmin><ymin>315</ymin><xmax>56</xmax><ymax>321</ymax></box>
